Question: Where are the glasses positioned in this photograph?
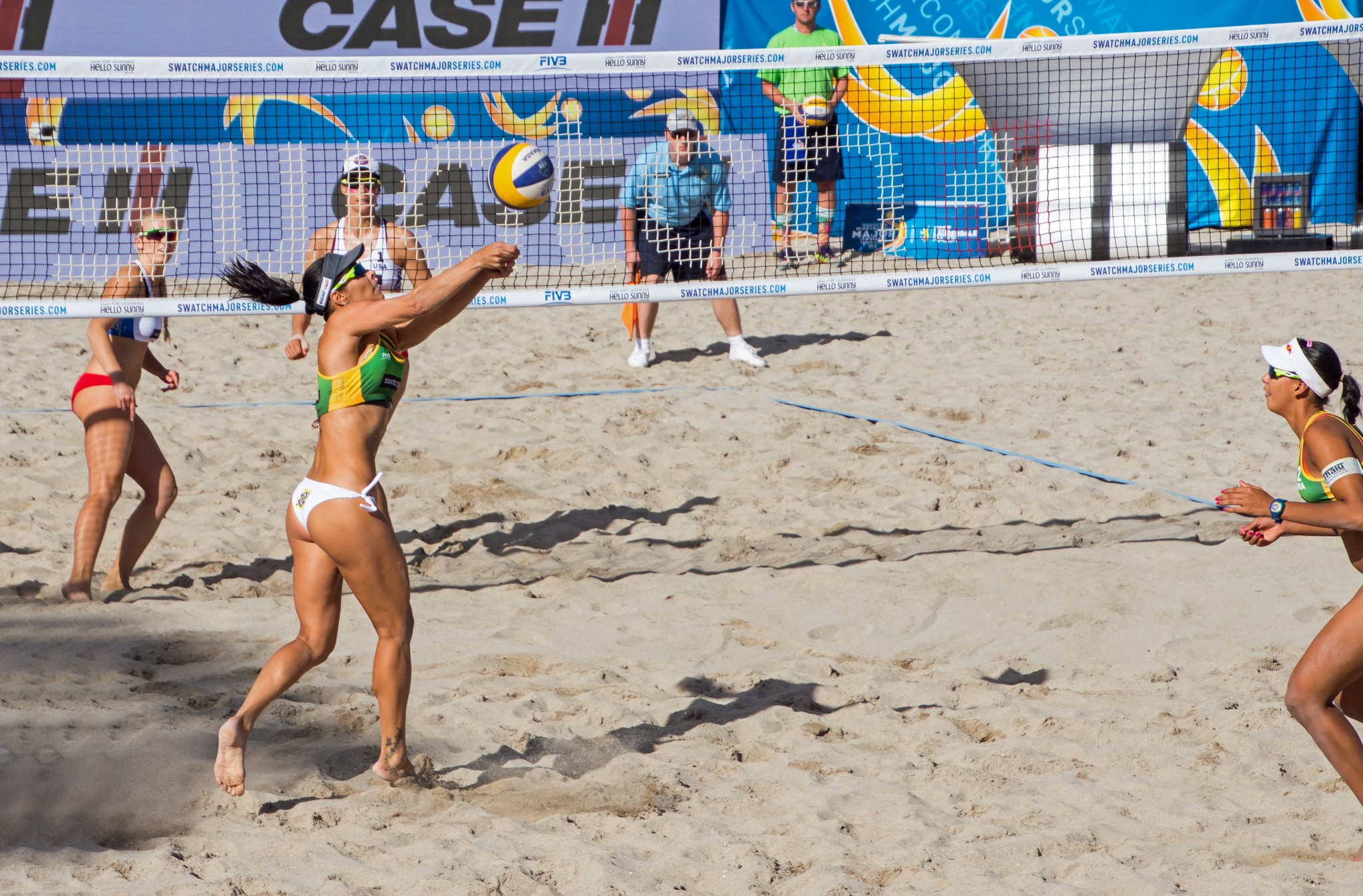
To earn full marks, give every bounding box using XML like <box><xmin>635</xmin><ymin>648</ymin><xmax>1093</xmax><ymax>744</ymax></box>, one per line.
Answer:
<box><xmin>136</xmin><ymin>228</ymin><xmax>177</xmax><ymax>241</ymax></box>
<box><xmin>665</xmin><ymin>128</ymin><xmax>698</xmax><ymax>139</ymax></box>
<box><xmin>793</xmin><ymin>0</ymin><xmax>819</xmax><ymax>8</ymax></box>
<box><xmin>330</xmin><ymin>262</ymin><xmax>366</xmax><ymax>294</ymax></box>
<box><xmin>341</xmin><ymin>177</ymin><xmax>383</xmax><ymax>194</ymax></box>
<box><xmin>1268</xmin><ymin>366</ymin><xmax>1302</xmax><ymax>379</ymax></box>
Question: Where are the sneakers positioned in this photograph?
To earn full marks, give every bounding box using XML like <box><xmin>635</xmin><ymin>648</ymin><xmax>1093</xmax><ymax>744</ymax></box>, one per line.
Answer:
<box><xmin>627</xmin><ymin>346</ymin><xmax>651</xmax><ymax>367</ymax></box>
<box><xmin>814</xmin><ymin>243</ymin><xmax>845</xmax><ymax>267</ymax></box>
<box><xmin>729</xmin><ymin>342</ymin><xmax>766</xmax><ymax>368</ymax></box>
<box><xmin>777</xmin><ymin>247</ymin><xmax>793</xmax><ymax>269</ymax></box>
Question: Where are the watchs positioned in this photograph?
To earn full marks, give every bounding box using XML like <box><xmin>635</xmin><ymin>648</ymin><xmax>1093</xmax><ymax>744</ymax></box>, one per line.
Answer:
<box><xmin>1269</xmin><ymin>497</ymin><xmax>1288</xmax><ymax>525</ymax></box>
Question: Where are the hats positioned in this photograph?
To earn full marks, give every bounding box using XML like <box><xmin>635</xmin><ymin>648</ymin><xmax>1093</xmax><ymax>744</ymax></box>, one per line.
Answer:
<box><xmin>342</xmin><ymin>153</ymin><xmax>381</xmax><ymax>180</ymax></box>
<box><xmin>314</xmin><ymin>242</ymin><xmax>365</xmax><ymax>317</ymax></box>
<box><xmin>666</xmin><ymin>106</ymin><xmax>699</xmax><ymax>131</ymax></box>
<box><xmin>1261</xmin><ymin>337</ymin><xmax>1334</xmax><ymax>398</ymax></box>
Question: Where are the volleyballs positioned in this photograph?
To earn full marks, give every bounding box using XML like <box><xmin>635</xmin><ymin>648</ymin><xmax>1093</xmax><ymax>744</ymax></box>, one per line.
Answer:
<box><xmin>488</xmin><ymin>143</ymin><xmax>555</xmax><ymax>210</ymax></box>
<box><xmin>799</xmin><ymin>95</ymin><xmax>831</xmax><ymax>127</ymax></box>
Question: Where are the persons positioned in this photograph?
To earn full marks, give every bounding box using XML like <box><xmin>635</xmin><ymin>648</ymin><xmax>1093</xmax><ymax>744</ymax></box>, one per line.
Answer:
<box><xmin>216</xmin><ymin>242</ymin><xmax>521</xmax><ymax>796</ymax></box>
<box><xmin>619</xmin><ymin>108</ymin><xmax>766</xmax><ymax>369</ymax></box>
<box><xmin>285</xmin><ymin>153</ymin><xmax>434</xmax><ymax>360</ymax></box>
<box><xmin>755</xmin><ymin>0</ymin><xmax>849</xmax><ymax>270</ymax></box>
<box><xmin>1215</xmin><ymin>336</ymin><xmax>1363</xmax><ymax>864</ymax></box>
<box><xmin>60</xmin><ymin>208</ymin><xmax>179</xmax><ymax>601</ymax></box>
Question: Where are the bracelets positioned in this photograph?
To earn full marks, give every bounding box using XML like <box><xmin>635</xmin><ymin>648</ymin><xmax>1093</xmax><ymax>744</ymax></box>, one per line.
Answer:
<box><xmin>712</xmin><ymin>245</ymin><xmax>723</xmax><ymax>254</ymax></box>
